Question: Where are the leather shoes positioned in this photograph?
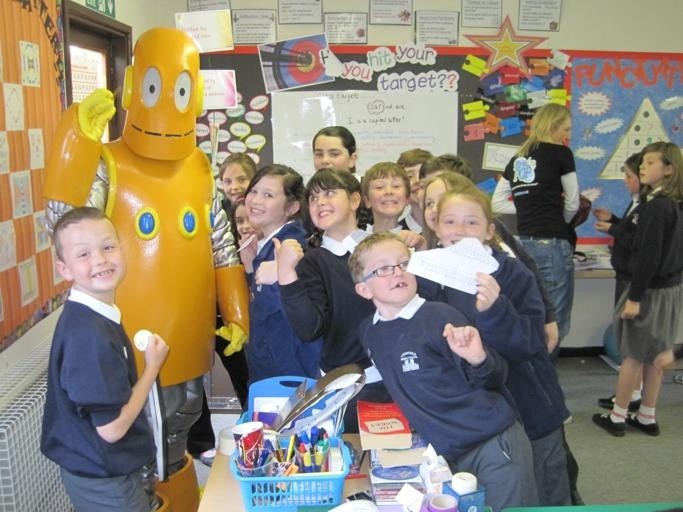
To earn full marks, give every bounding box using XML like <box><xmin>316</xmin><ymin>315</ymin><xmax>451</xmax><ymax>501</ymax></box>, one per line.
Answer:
<box><xmin>592</xmin><ymin>394</ymin><xmax>660</xmax><ymax>437</ymax></box>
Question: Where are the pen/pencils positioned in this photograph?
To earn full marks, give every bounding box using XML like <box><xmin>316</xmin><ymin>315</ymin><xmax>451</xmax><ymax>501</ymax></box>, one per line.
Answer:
<box><xmin>238</xmin><ymin>426</ymin><xmax>328</xmax><ymax>502</ymax></box>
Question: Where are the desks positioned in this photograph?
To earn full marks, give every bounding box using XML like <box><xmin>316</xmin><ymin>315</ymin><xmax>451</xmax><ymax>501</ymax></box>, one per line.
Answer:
<box><xmin>194</xmin><ymin>431</ymin><xmax>377</xmax><ymax>512</ymax></box>
<box><xmin>556</xmin><ymin>267</ymin><xmax>682</xmax><ymax>349</ymax></box>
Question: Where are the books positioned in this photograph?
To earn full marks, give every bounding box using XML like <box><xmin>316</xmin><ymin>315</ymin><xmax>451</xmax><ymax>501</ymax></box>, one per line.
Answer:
<box><xmin>357</xmin><ymin>399</ymin><xmax>412</xmax><ymax>450</ymax></box>
<box><xmin>367</xmin><ymin>466</ymin><xmax>425</xmax><ymax>505</ymax></box>
<box><xmin>329</xmin><ymin>447</ymin><xmax>342</xmax><ymax>472</ymax></box>
<box><xmin>370</xmin><ymin>448</ymin><xmax>381</xmax><ymax>468</ymax></box>
<box><xmin>378</xmin><ymin>431</ymin><xmax>428</xmax><ymax>469</ymax></box>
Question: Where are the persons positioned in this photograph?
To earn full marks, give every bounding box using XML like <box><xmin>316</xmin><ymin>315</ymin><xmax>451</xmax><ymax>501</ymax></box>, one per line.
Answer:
<box><xmin>215</xmin><ymin>153</ymin><xmax>324</xmax><ymax>409</ymax></box>
<box><xmin>592</xmin><ymin>141</ymin><xmax>682</xmax><ymax>438</ymax></box>
<box><xmin>308</xmin><ymin>126</ymin><xmax>374</xmax><ymax>247</ymax></box>
<box><xmin>349</xmin><ymin>232</ymin><xmax>540</xmax><ymax>511</ymax></box>
<box><xmin>491</xmin><ymin>103</ymin><xmax>579</xmax><ymax>366</ymax></box>
<box><xmin>272</xmin><ymin>168</ymin><xmax>393</xmax><ymax>434</ymax></box>
<box><xmin>39</xmin><ymin>207</ymin><xmax>170</xmax><ymax>512</ymax></box>
<box><xmin>436</xmin><ymin>187</ymin><xmax>573</xmax><ymax>505</ymax></box>
<box><xmin>363</xmin><ymin>149</ymin><xmax>583</xmax><ymax>507</ymax></box>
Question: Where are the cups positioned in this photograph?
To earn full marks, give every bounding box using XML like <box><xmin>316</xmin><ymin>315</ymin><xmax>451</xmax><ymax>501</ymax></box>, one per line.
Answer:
<box><xmin>294</xmin><ymin>441</ymin><xmax>330</xmax><ymax>471</ymax></box>
<box><xmin>427</xmin><ymin>493</ymin><xmax>458</xmax><ymax>511</ymax></box>
<box><xmin>230</xmin><ymin>420</ymin><xmax>263</xmax><ymax>462</ymax></box>
<box><xmin>235</xmin><ymin>446</ymin><xmax>274</xmax><ymax>476</ymax></box>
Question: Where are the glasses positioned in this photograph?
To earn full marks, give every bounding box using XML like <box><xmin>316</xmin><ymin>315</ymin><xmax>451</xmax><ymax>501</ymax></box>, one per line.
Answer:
<box><xmin>360</xmin><ymin>261</ymin><xmax>408</xmax><ymax>284</ymax></box>
